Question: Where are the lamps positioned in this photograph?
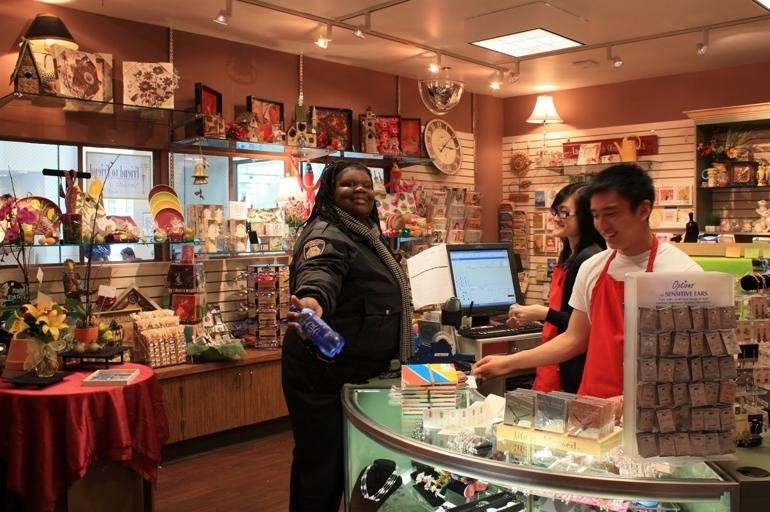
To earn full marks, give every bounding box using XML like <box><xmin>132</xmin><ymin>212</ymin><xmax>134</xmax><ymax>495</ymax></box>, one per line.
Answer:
<box><xmin>523</xmin><ymin>91</ymin><xmax>565</xmax><ymax>168</ymax></box>
<box><xmin>503</xmin><ymin>22</ymin><xmax>716</xmax><ymax>86</ymax></box>
<box><xmin>213</xmin><ymin>0</ymin><xmax>510</xmax><ymax>91</ymax></box>
<box><xmin>414</xmin><ymin>63</ymin><xmax>465</xmax><ymax>117</ymax></box>
<box><xmin>13</xmin><ymin>11</ymin><xmax>82</xmax><ymax>95</ymax></box>
<box><xmin>350</xmin><ymin>11</ymin><xmax>374</xmax><ymax>40</ymax></box>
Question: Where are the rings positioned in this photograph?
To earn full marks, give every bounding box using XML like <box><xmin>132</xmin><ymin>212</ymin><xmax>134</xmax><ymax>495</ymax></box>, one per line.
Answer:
<box><xmin>514</xmin><ymin>312</ymin><xmax>522</xmax><ymax>320</ymax></box>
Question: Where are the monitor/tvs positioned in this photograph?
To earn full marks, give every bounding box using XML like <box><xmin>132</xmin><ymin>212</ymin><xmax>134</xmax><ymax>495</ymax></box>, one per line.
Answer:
<box><xmin>407</xmin><ymin>243</ymin><xmax>524</xmax><ymax>330</ymax></box>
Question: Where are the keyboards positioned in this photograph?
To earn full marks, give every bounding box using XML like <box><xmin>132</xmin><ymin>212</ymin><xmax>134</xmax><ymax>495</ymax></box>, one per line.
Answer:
<box><xmin>458</xmin><ymin>322</ymin><xmax>544</xmax><ymax>339</ymax></box>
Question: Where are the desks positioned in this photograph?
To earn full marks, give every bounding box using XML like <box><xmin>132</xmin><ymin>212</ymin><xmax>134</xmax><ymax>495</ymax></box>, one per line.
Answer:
<box><xmin>0</xmin><ymin>358</ymin><xmax>169</xmax><ymax>512</ymax></box>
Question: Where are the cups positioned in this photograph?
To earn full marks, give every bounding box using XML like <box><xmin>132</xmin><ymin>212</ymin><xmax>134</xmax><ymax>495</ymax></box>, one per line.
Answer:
<box><xmin>250</xmin><ymin>223</ymin><xmax>289</xmax><ymax>236</ymax></box>
<box><xmin>701</xmin><ymin>167</ymin><xmax>729</xmax><ymax>188</ymax></box>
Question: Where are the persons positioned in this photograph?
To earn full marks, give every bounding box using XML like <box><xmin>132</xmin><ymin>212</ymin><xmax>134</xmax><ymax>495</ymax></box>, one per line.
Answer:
<box><xmin>504</xmin><ymin>181</ymin><xmax>608</xmax><ymax>393</ymax></box>
<box><xmin>279</xmin><ymin>154</ymin><xmax>418</xmax><ymax>510</ymax></box>
<box><xmin>463</xmin><ymin>160</ymin><xmax>707</xmax><ymax>427</ymax></box>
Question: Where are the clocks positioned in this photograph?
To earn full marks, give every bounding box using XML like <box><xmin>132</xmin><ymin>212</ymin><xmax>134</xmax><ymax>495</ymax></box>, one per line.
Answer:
<box><xmin>422</xmin><ymin>117</ymin><xmax>464</xmax><ymax>177</ymax></box>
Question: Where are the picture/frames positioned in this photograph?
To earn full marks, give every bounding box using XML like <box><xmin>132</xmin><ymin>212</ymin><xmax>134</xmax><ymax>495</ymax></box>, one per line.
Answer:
<box><xmin>194</xmin><ymin>80</ymin><xmax>224</xmax><ymax>134</ymax></box>
<box><xmin>308</xmin><ymin>103</ymin><xmax>355</xmax><ymax>152</ymax></box>
<box><xmin>141</xmin><ymin>210</ymin><xmax>154</xmax><ymax>238</ymax></box>
<box><xmin>245</xmin><ymin>92</ymin><xmax>286</xmax><ymax>143</ymax></box>
<box><xmin>355</xmin><ymin>112</ymin><xmax>403</xmax><ymax>155</ymax></box>
<box><xmin>397</xmin><ymin>114</ymin><xmax>425</xmax><ymax>156</ymax></box>
<box><xmin>576</xmin><ymin>141</ymin><xmax>602</xmax><ymax>166</ymax></box>
<box><xmin>85</xmin><ymin>151</ymin><xmax>152</xmax><ymax>200</ymax></box>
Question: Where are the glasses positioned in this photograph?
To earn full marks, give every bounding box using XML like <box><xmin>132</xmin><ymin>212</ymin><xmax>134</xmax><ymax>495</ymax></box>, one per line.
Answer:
<box><xmin>549</xmin><ymin>206</ymin><xmax>578</xmax><ymax>219</ymax></box>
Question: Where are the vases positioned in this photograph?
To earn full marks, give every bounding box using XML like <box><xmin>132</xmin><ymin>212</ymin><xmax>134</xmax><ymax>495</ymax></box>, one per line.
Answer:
<box><xmin>700</xmin><ymin>163</ymin><xmax>729</xmax><ymax>187</ymax></box>
<box><xmin>2</xmin><ymin>333</ymin><xmax>61</xmax><ymax>380</ymax></box>
<box><xmin>73</xmin><ymin>325</ymin><xmax>100</xmax><ymax>344</ymax></box>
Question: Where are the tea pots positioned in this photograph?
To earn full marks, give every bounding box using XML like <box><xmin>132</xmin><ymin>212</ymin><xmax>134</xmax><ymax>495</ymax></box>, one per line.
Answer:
<box><xmin>614</xmin><ymin>134</ymin><xmax>641</xmax><ymax>161</ymax></box>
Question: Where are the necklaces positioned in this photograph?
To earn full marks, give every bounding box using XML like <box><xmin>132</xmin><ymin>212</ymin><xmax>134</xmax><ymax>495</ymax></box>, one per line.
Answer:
<box><xmin>357</xmin><ymin>462</ymin><xmax>400</xmax><ymax>502</ymax></box>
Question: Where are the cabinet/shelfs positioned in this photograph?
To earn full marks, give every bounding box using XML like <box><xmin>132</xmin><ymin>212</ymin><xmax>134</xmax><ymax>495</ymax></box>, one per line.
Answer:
<box><xmin>170</xmin><ymin>136</ymin><xmax>436</xmax><ymax>259</ymax></box>
<box><xmin>337</xmin><ymin>378</ymin><xmax>745</xmax><ymax>512</ymax></box>
<box><xmin>680</xmin><ymin>98</ymin><xmax>770</xmax><ymax>244</ymax></box>
<box><xmin>151</xmin><ymin>362</ymin><xmax>184</xmax><ymax>450</ymax></box>
<box><xmin>182</xmin><ymin>346</ymin><xmax>291</xmax><ymax>442</ymax></box>
<box><xmin>1</xmin><ymin>90</ymin><xmax>205</xmax><ymax>252</ymax></box>
<box><xmin>450</xmin><ymin>329</ymin><xmax>549</xmax><ymax>397</ymax></box>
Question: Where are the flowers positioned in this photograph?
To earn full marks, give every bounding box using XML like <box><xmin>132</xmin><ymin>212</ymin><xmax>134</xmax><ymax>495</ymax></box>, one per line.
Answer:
<box><xmin>58</xmin><ymin>151</ymin><xmax>153</xmax><ymax>326</ymax></box>
<box><xmin>696</xmin><ymin>126</ymin><xmax>765</xmax><ymax>161</ymax></box>
<box><xmin>0</xmin><ymin>163</ymin><xmax>71</xmax><ymax>372</ymax></box>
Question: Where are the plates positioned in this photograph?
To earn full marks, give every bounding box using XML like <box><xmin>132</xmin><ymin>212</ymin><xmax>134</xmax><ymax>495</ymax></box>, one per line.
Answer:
<box><xmin>12</xmin><ymin>195</ymin><xmax>62</xmax><ymax>235</ymax></box>
<box><xmin>149</xmin><ymin>184</ymin><xmax>189</xmax><ymax>242</ymax></box>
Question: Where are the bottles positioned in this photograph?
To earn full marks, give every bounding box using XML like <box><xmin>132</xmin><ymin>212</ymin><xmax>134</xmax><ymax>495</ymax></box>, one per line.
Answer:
<box><xmin>684</xmin><ymin>212</ymin><xmax>699</xmax><ymax>243</ymax></box>
<box><xmin>290</xmin><ymin>303</ymin><xmax>346</xmax><ymax>360</ymax></box>
